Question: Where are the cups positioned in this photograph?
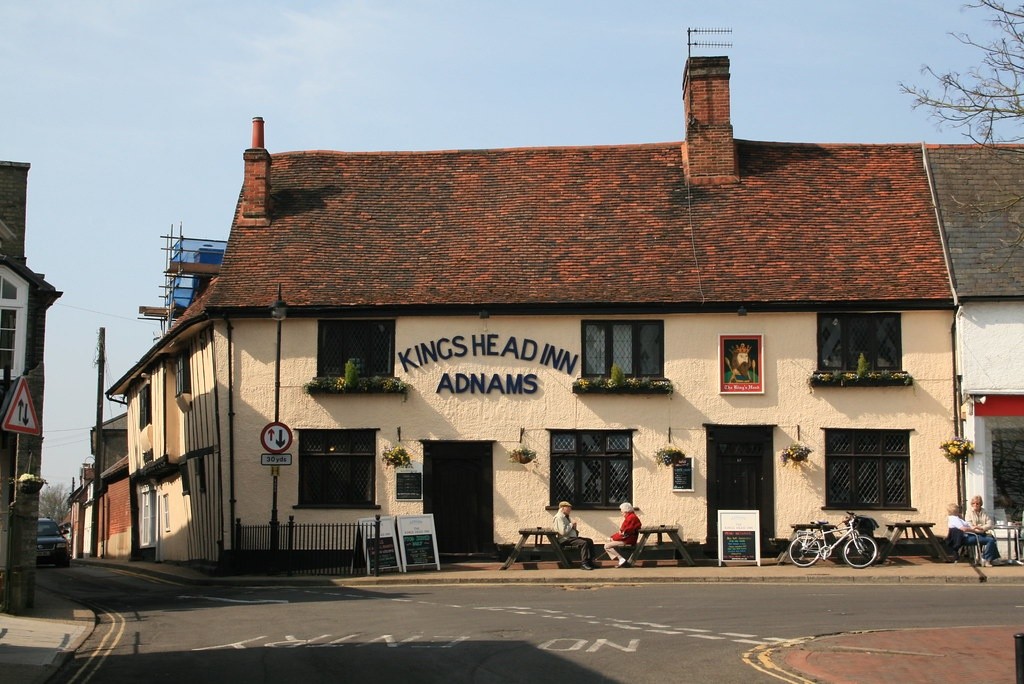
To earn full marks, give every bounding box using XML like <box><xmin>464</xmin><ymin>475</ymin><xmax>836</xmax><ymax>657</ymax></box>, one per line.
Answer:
<box><xmin>572</xmin><ymin>520</ymin><xmax>576</xmax><ymax>530</ymax></box>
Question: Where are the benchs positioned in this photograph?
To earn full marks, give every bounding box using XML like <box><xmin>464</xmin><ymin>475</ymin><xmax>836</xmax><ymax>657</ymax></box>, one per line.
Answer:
<box><xmin>497</xmin><ymin>541</ymin><xmax>701</xmax><ymax>552</ymax></box>
<box><xmin>768</xmin><ymin>537</ymin><xmax>945</xmax><ymax>550</ymax></box>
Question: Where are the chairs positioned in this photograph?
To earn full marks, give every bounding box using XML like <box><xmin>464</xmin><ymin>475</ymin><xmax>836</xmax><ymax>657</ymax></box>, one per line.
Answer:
<box><xmin>949</xmin><ymin>528</ymin><xmax>984</xmax><ymax>566</ymax></box>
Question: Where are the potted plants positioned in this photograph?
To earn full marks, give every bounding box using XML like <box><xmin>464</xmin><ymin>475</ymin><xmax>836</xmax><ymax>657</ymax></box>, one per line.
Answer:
<box><xmin>654</xmin><ymin>448</ymin><xmax>687</xmax><ymax>466</ymax></box>
<box><xmin>381</xmin><ymin>446</ymin><xmax>413</xmax><ymax>469</ymax></box>
<box><xmin>571</xmin><ymin>364</ymin><xmax>673</xmax><ymax>397</ymax></box>
<box><xmin>508</xmin><ymin>448</ymin><xmax>535</xmax><ymax>465</ymax></box>
<box><xmin>807</xmin><ymin>354</ymin><xmax>914</xmax><ymax>386</ymax></box>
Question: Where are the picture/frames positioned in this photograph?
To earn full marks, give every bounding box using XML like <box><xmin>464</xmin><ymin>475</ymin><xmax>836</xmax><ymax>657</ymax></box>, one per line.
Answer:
<box><xmin>717</xmin><ymin>333</ymin><xmax>765</xmax><ymax>395</ymax></box>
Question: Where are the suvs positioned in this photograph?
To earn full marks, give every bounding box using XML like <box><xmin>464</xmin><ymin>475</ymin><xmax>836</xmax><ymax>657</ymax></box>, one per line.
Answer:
<box><xmin>38</xmin><ymin>515</ymin><xmax>70</xmax><ymax>569</ymax></box>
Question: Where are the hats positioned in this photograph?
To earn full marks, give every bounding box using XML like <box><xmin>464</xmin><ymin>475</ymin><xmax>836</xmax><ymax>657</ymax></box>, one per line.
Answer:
<box><xmin>559</xmin><ymin>501</ymin><xmax>572</xmax><ymax>507</ymax></box>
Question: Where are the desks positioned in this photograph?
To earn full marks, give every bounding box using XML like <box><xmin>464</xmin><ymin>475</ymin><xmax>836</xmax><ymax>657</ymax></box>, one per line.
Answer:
<box><xmin>624</xmin><ymin>525</ymin><xmax>696</xmax><ymax>566</ymax></box>
<box><xmin>983</xmin><ymin>525</ymin><xmax>1021</xmax><ymax>565</ymax></box>
<box><xmin>499</xmin><ymin>527</ymin><xmax>571</xmax><ymax>570</ymax></box>
<box><xmin>877</xmin><ymin>521</ymin><xmax>951</xmax><ymax>564</ymax></box>
<box><xmin>779</xmin><ymin>524</ymin><xmax>846</xmax><ymax>563</ymax></box>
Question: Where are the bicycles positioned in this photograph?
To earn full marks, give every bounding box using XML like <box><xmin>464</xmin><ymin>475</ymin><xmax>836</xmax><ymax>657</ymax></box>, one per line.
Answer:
<box><xmin>788</xmin><ymin>511</ymin><xmax>878</xmax><ymax>568</ymax></box>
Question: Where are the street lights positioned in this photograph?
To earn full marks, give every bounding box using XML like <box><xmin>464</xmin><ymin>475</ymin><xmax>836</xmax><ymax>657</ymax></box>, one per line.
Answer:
<box><xmin>270</xmin><ymin>281</ymin><xmax>287</xmax><ymax>572</ymax></box>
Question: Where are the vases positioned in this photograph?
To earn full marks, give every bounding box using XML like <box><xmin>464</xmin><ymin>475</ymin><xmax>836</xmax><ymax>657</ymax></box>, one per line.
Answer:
<box><xmin>789</xmin><ymin>455</ymin><xmax>804</xmax><ymax>462</ymax></box>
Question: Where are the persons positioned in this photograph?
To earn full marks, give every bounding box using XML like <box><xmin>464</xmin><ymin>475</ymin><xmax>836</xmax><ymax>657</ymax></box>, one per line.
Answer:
<box><xmin>552</xmin><ymin>501</ymin><xmax>600</xmax><ymax>569</ymax></box>
<box><xmin>947</xmin><ymin>495</ymin><xmax>1001</xmax><ymax>567</ymax></box>
<box><xmin>603</xmin><ymin>502</ymin><xmax>642</xmax><ymax>569</ymax></box>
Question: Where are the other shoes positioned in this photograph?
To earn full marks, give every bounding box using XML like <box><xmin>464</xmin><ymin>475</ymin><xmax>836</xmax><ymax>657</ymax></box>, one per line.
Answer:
<box><xmin>615</xmin><ymin>560</ymin><xmax>627</xmax><ymax>568</ymax></box>
<box><xmin>588</xmin><ymin>561</ymin><xmax>599</xmax><ymax>568</ymax></box>
<box><xmin>581</xmin><ymin>562</ymin><xmax>592</xmax><ymax>570</ymax></box>
<box><xmin>980</xmin><ymin>560</ymin><xmax>992</xmax><ymax>568</ymax></box>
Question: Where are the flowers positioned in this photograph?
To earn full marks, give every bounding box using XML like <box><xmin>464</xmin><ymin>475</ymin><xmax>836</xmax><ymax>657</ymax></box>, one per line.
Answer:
<box><xmin>780</xmin><ymin>443</ymin><xmax>812</xmax><ymax>466</ymax></box>
<box><xmin>940</xmin><ymin>436</ymin><xmax>975</xmax><ymax>462</ymax></box>
